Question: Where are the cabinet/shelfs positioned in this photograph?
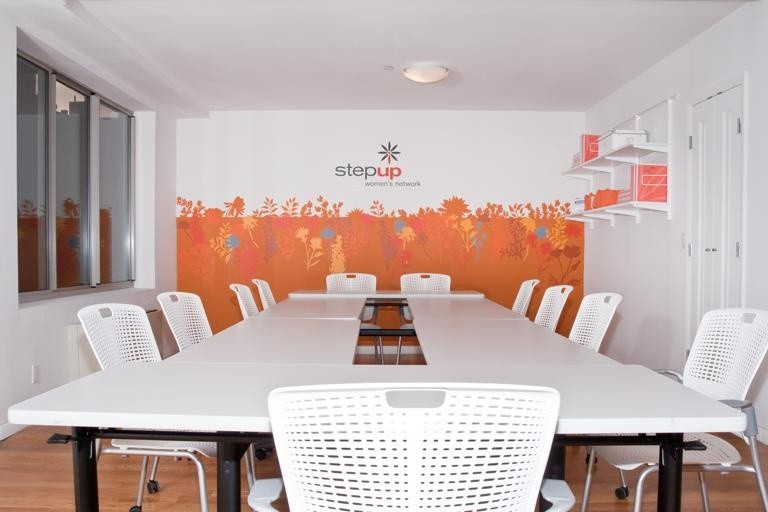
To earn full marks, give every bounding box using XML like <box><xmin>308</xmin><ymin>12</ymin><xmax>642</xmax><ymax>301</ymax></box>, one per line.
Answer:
<box><xmin>559</xmin><ymin>94</ymin><xmax>676</xmax><ymax>227</ymax></box>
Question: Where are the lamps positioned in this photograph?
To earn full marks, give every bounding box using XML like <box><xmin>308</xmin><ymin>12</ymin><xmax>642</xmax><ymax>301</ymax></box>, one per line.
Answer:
<box><xmin>401</xmin><ymin>65</ymin><xmax>449</xmax><ymax>86</ymax></box>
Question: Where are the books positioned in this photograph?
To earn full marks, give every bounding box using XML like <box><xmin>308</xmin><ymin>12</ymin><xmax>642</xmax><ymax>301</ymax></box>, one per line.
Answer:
<box><xmin>571</xmin><ymin>131</ymin><xmax>602</xmax><ymax>166</ymax></box>
<box><xmin>574</xmin><ymin>162</ymin><xmax>670</xmax><ymax>211</ymax></box>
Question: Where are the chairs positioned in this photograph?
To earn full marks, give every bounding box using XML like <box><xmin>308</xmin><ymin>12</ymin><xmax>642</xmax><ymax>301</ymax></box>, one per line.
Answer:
<box><xmin>156</xmin><ymin>290</ymin><xmax>215</xmax><ymax>350</ymax></box>
<box><xmin>535</xmin><ymin>285</ymin><xmax>576</xmax><ymax>337</ymax></box>
<box><xmin>251</xmin><ymin>275</ymin><xmax>276</xmax><ymax>314</ymax></box>
<box><xmin>580</xmin><ymin>306</ymin><xmax>768</xmax><ymax>508</ymax></box>
<box><xmin>511</xmin><ymin>273</ymin><xmax>540</xmax><ymax>316</ymax></box>
<box><xmin>230</xmin><ymin>280</ymin><xmax>259</xmax><ymax>322</ymax></box>
<box><xmin>324</xmin><ymin>273</ymin><xmax>377</xmax><ymax>293</ymax></box>
<box><xmin>397</xmin><ymin>269</ymin><xmax>454</xmax><ymax>290</ymax></box>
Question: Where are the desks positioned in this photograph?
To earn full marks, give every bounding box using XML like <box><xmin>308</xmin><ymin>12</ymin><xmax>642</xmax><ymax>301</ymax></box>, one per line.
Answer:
<box><xmin>15</xmin><ymin>292</ymin><xmax>746</xmax><ymax>508</ymax></box>
